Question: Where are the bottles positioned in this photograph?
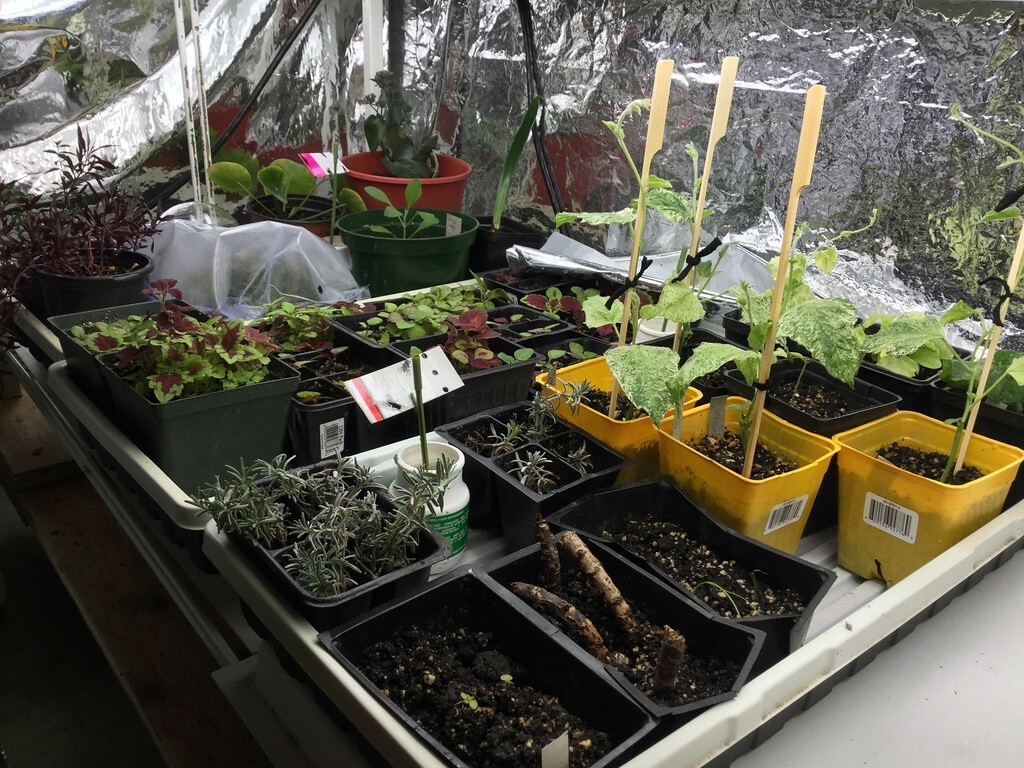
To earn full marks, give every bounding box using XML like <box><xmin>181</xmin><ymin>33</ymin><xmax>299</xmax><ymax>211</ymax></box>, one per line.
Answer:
<box><xmin>388</xmin><ymin>440</ymin><xmax>471</xmax><ymax>575</ymax></box>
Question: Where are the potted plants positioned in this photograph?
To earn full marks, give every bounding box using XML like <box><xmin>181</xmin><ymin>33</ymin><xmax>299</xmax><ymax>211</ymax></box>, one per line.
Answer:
<box><xmin>2</xmin><ymin>69</ymin><xmax>1024</xmax><ymax>768</ymax></box>
